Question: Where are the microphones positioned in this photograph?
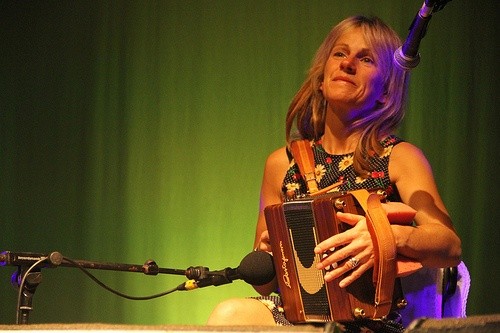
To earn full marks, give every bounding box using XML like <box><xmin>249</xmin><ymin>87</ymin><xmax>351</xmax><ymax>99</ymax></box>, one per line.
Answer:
<box><xmin>394</xmin><ymin>2</ymin><xmax>440</xmax><ymax>70</ymax></box>
<box><xmin>177</xmin><ymin>249</ymin><xmax>275</xmax><ymax>291</ymax></box>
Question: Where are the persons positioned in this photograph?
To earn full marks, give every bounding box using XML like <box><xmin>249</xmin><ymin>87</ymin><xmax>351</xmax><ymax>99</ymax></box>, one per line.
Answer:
<box><xmin>251</xmin><ymin>11</ymin><xmax>461</xmax><ymax>332</ymax></box>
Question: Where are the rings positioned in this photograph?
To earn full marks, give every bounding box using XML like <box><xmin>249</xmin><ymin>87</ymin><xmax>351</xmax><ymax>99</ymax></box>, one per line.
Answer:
<box><xmin>346</xmin><ymin>257</ymin><xmax>361</xmax><ymax>270</ymax></box>
